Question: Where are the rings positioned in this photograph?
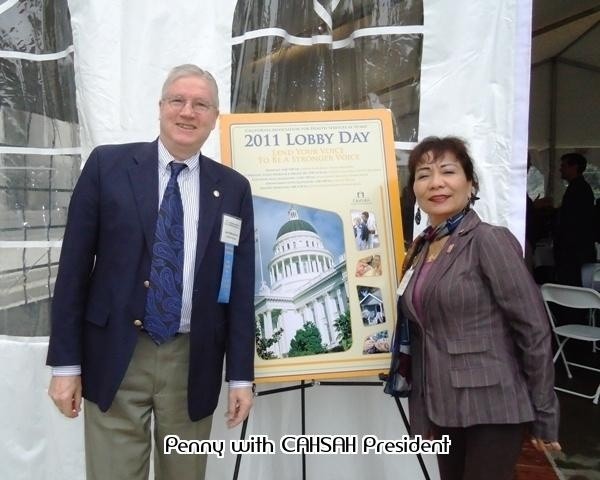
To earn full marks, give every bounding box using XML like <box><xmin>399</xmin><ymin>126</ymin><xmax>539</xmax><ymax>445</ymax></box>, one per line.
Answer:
<box><xmin>542</xmin><ymin>440</ymin><xmax>553</xmax><ymax>446</ymax></box>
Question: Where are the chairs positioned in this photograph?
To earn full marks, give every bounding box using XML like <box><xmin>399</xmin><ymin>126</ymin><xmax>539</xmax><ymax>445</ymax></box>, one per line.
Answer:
<box><xmin>539</xmin><ymin>281</ymin><xmax>600</xmax><ymax>405</ymax></box>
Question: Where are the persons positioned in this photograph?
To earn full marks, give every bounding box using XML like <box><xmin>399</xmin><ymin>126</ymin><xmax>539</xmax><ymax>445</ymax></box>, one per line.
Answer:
<box><xmin>46</xmin><ymin>60</ymin><xmax>256</xmax><ymax>480</ymax></box>
<box><xmin>354</xmin><ymin>217</ymin><xmax>368</xmax><ymax>249</ymax></box>
<box><xmin>551</xmin><ymin>154</ymin><xmax>599</xmax><ymax>366</ymax></box>
<box><xmin>526</xmin><ymin>152</ymin><xmax>555</xmax><ymax>279</ymax></box>
<box><xmin>372</xmin><ymin>255</ymin><xmax>382</xmax><ymax>277</ymax></box>
<box><xmin>361</xmin><ymin>211</ymin><xmax>376</xmax><ymax>248</ymax></box>
<box><xmin>389</xmin><ymin>133</ymin><xmax>565</xmax><ymax>480</ymax></box>
<box><xmin>356</xmin><ymin>259</ymin><xmax>368</xmax><ymax>277</ymax></box>
<box><xmin>362</xmin><ymin>336</ymin><xmax>391</xmax><ymax>354</ymax></box>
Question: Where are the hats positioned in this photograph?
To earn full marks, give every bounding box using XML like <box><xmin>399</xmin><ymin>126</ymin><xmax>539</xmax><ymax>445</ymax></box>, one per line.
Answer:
<box><xmin>560</xmin><ymin>153</ymin><xmax>587</xmax><ymax>169</ymax></box>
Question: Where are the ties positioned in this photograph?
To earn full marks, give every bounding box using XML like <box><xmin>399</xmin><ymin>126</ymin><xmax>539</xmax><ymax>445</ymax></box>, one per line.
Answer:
<box><xmin>143</xmin><ymin>162</ymin><xmax>188</xmax><ymax>346</ymax></box>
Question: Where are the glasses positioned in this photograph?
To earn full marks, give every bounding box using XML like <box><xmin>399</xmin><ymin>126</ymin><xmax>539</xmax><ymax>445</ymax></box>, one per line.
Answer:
<box><xmin>168</xmin><ymin>94</ymin><xmax>212</xmax><ymax>113</ymax></box>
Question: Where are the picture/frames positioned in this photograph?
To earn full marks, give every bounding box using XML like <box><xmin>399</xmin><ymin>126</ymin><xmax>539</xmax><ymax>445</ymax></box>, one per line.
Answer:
<box><xmin>218</xmin><ymin>109</ymin><xmax>407</xmax><ymax>385</ymax></box>
